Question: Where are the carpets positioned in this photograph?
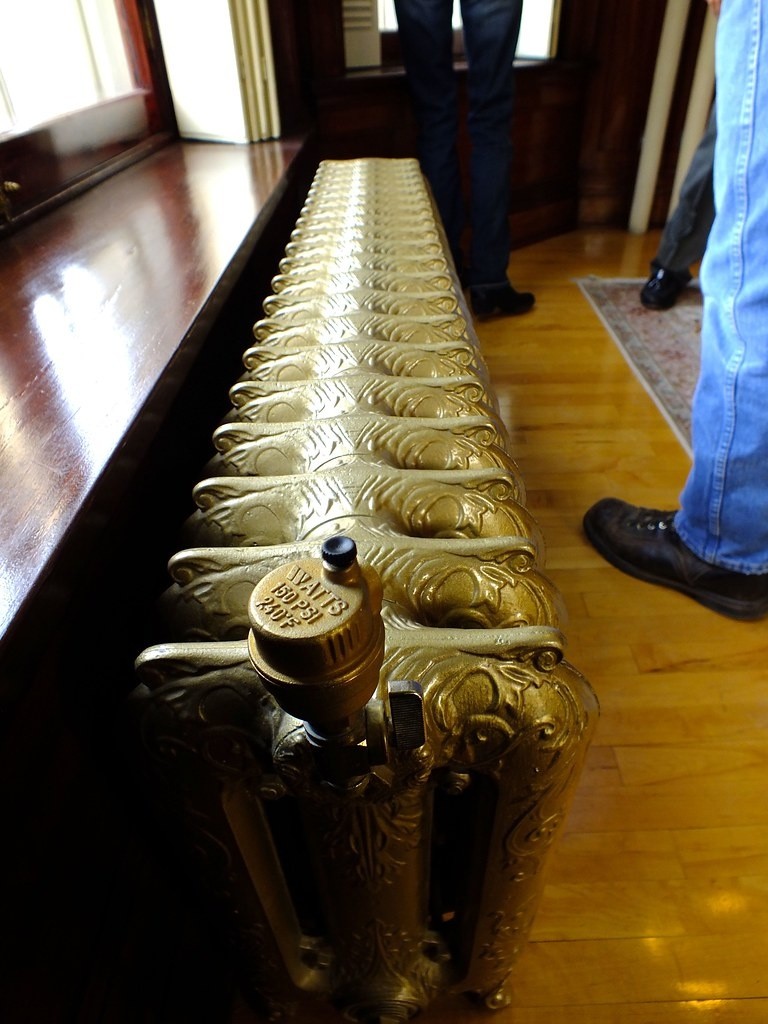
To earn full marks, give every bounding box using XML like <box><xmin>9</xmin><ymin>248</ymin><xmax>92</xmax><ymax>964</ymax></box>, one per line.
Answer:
<box><xmin>574</xmin><ymin>276</ymin><xmax>706</xmax><ymax>467</ymax></box>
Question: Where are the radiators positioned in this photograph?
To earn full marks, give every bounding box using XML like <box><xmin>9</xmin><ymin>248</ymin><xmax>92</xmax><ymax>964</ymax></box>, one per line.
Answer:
<box><xmin>136</xmin><ymin>154</ymin><xmax>603</xmax><ymax>1024</ymax></box>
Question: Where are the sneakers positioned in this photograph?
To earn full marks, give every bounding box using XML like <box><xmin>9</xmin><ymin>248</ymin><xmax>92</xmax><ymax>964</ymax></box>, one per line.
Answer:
<box><xmin>582</xmin><ymin>496</ymin><xmax>768</xmax><ymax>617</ymax></box>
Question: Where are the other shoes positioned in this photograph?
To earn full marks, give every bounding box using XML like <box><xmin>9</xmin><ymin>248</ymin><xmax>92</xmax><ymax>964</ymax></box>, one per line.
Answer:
<box><xmin>471</xmin><ymin>284</ymin><xmax>536</xmax><ymax>320</ymax></box>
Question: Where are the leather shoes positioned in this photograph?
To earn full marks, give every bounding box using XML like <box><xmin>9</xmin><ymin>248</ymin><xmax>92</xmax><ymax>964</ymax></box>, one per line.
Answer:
<box><xmin>640</xmin><ymin>258</ymin><xmax>688</xmax><ymax>309</ymax></box>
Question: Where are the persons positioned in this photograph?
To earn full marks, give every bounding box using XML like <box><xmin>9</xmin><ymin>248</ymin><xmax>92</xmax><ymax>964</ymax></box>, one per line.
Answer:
<box><xmin>393</xmin><ymin>0</ymin><xmax>535</xmax><ymax>321</ymax></box>
<box><xmin>583</xmin><ymin>1</ymin><xmax>768</xmax><ymax>621</ymax></box>
<box><xmin>639</xmin><ymin>96</ymin><xmax>719</xmax><ymax>310</ymax></box>
